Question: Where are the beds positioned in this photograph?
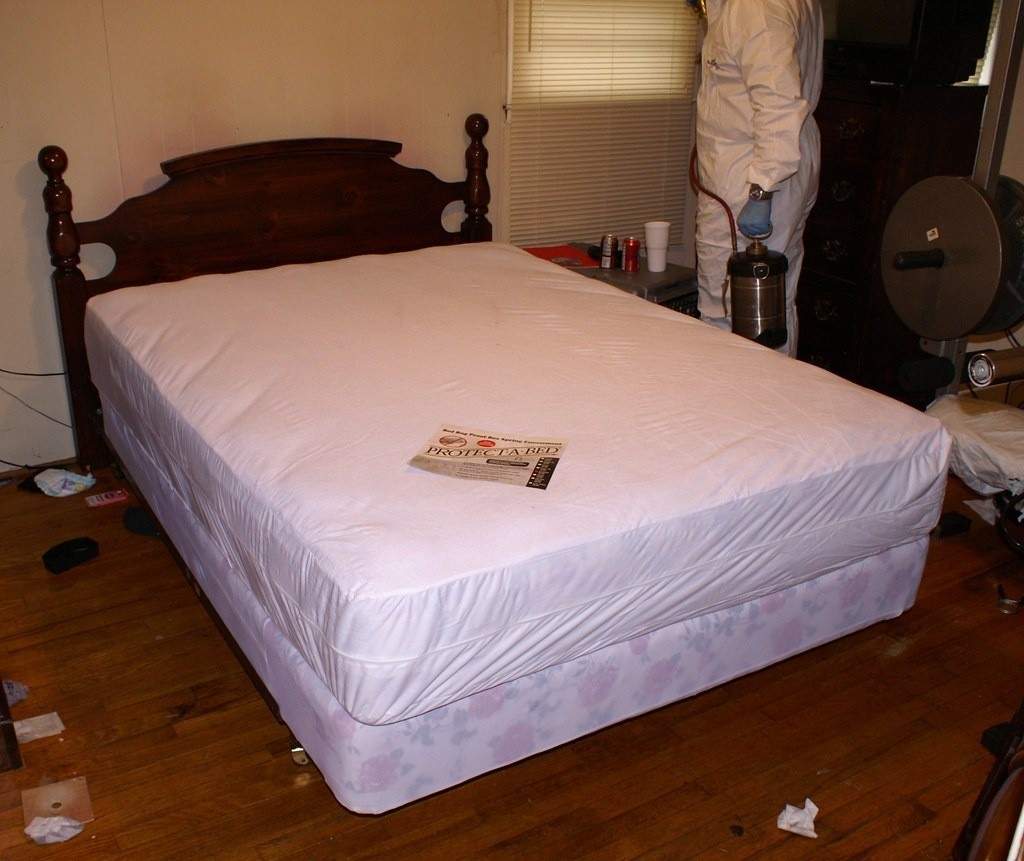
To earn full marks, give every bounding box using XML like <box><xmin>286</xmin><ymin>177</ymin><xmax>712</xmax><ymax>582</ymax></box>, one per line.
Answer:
<box><xmin>38</xmin><ymin>112</ymin><xmax>955</xmax><ymax>816</ymax></box>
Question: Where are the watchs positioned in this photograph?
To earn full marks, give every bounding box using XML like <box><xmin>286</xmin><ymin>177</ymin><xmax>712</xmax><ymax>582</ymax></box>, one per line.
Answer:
<box><xmin>749</xmin><ymin>184</ymin><xmax>774</xmax><ymax>204</ymax></box>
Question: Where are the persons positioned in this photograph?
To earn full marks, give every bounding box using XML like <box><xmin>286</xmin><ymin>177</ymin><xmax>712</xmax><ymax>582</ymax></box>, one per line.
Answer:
<box><xmin>696</xmin><ymin>1</ymin><xmax>823</xmax><ymax>358</ymax></box>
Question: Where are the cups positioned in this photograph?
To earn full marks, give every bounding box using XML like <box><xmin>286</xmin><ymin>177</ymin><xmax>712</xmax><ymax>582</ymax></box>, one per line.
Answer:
<box><xmin>644</xmin><ymin>221</ymin><xmax>671</xmax><ymax>272</ymax></box>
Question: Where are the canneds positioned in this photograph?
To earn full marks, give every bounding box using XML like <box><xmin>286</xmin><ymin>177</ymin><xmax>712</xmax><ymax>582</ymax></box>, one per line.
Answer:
<box><xmin>600</xmin><ymin>234</ymin><xmax>618</xmax><ymax>269</ymax></box>
<box><xmin>622</xmin><ymin>237</ymin><xmax>640</xmax><ymax>273</ymax></box>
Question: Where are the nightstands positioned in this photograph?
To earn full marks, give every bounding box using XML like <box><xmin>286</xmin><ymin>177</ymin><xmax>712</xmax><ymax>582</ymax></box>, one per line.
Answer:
<box><xmin>568</xmin><ymin>258</ymin><xmax>699</xmax><ymax>318</ymax></box>
